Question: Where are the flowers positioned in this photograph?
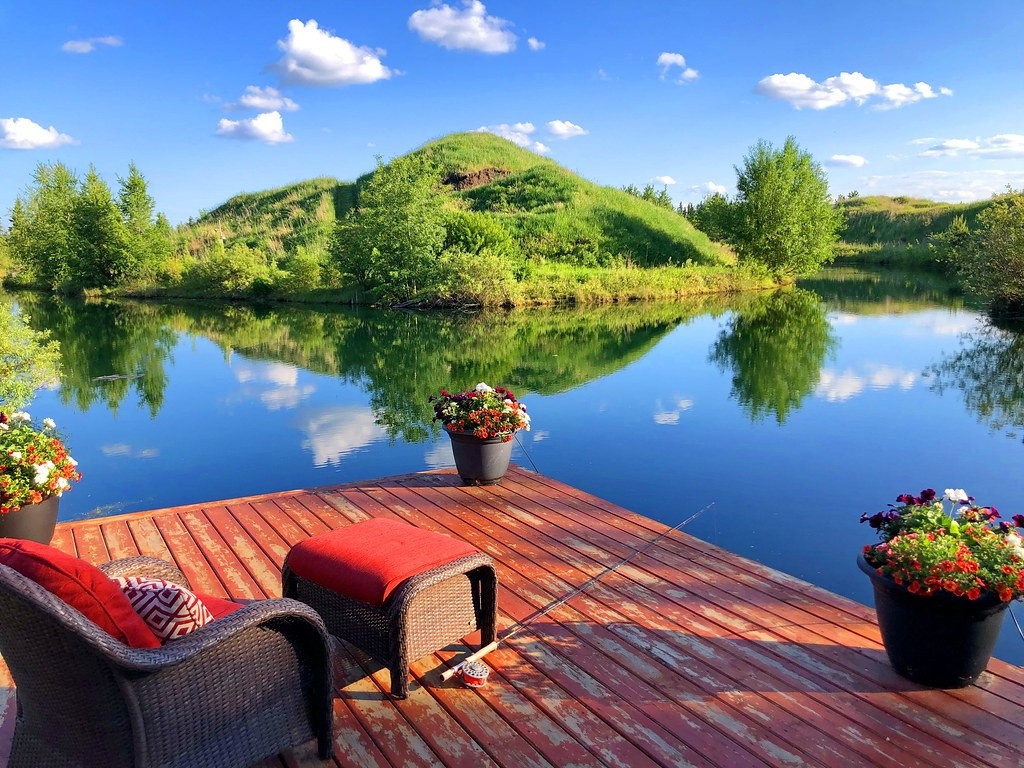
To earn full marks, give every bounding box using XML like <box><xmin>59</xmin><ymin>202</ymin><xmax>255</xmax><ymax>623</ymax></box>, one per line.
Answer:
<box><xmin>860</xmin><ymin>487</ymin><xmax>1023</xmax><ymax>603</ymax></box>
<box><xmin>426</xmin><ymin>383</ymin><xmax>532</xmax><ymax>446</ymax></box>
<box><xmin>0</xmin><ymin>410</ymin><xmax>82</xmax><ymax>514</ymax></box>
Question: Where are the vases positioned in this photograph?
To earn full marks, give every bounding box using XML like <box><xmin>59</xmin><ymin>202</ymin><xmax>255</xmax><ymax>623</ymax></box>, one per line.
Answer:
<box><xmin>0</xmin><ymin>494</ymin><xmax>59</xmax><ymax>545</ymax></box>
<box><xmin>856</xmin><ymin>539</ymin><xmax>1009</xmax><ymax>690</ymax></box>
<box><xmin>441</xmin><ymin>422</ymin><xmax>515</xmax><ymax>486</ymax></box>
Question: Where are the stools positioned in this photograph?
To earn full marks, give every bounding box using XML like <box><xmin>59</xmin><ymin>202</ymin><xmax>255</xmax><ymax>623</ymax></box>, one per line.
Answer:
<box><xmin>281</xmin><ymin>518</ymin><xmax>497</xmax><ymax>699</ymax></box>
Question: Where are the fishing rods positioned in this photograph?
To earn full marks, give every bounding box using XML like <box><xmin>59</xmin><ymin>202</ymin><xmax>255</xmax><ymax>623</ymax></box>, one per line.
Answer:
<box><xmin>440</xmin><ymin>501</ymin><xmax>715</xmax><ymax>687</ymax></box>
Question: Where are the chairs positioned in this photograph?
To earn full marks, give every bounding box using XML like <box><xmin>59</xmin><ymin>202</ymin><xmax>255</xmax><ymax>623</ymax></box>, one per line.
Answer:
<box><xmin>0</xmin><ymin>539</ymin><xmax>338</xmax><ymax>768</ymax></box>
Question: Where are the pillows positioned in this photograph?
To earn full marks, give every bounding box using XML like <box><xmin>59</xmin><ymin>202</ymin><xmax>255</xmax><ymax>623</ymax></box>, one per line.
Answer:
<box><xmin>0</xmin><ymin>537</ymin><xmax>166</xmax><ymax>649</ymax></box>
<box><xmin>113</xmin><ymin>571</ymin><xmax>216</xmax><ymax>643</ymax></box>
